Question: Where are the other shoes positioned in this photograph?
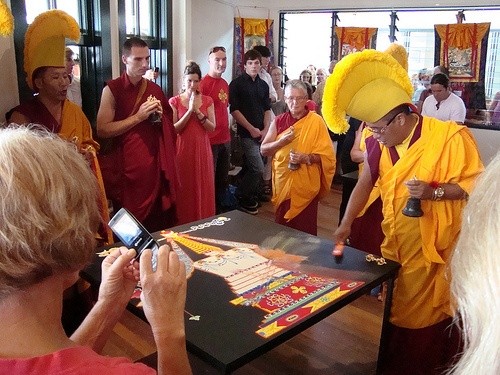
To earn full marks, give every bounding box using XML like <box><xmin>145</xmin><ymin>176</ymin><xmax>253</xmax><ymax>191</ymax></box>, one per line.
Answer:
<box><xmin>238</xmin><ymin>198</ymin><xmax>259</xmax><ymax>215</ymax></box>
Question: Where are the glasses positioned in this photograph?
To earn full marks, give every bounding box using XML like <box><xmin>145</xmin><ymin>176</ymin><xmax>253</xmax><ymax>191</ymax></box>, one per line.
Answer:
<box><xmin>368</xmin><ymin>111</ymin><xmax>404</xmax><ymax>134</ymax></box>
<box><xmin>209</xmin><ymin>46</ymin><xmax>226</xmax><ymax>56</ymax></box>
<box><xmin>286</xmin><ymin>94</ymin><xmax>307</xmax><ymax>102</ymax></box>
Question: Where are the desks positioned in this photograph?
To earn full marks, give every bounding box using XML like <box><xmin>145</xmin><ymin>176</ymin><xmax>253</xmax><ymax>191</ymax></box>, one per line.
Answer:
<box><xmin>79</xmin><ymin>210</ymin><xmax>403</xmax><ymax>375</ymax></box>
<box><xmin>341</xmin><ymin>170</ymin><xmax>389</xmax><ymax>255</ymax></box>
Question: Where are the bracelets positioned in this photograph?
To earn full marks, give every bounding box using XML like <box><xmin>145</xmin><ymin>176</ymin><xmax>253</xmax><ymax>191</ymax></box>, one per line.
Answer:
<box><xmin>200</xmin><ymin>115</ymin><xmax>207</xmax><ymax>121</ymax></box>
<box><xmin>201</xmin><ymin>118</ymin><xmax>206</xmax><ymax>125</ymax></box>
<box><xmin>196</xmin><ymin>111</ymin><xmax>202</xmax><ymax>115</ymax></box>
<box><xmin>306</xmin><ymin>155</ymin><xmax>314</xmax><ymax>166</ymax></box>
<box><xmin>430</xmin><ymin>180</ymin><xmax>438</xmax><ymax>200</ymax></box>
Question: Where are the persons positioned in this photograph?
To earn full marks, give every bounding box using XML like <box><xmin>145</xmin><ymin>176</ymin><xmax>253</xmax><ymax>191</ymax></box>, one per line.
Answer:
<box><xmin>0</xmin><ymin>9</ymin><xmax>500</xmax><ymax>375</ymax></box>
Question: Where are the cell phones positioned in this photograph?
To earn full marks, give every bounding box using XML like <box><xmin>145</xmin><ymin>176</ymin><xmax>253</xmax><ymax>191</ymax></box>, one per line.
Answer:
<box><xmin>108</xmin><ymin>207</ymin><xmax>160</xmax><ymax>272</ymax></box>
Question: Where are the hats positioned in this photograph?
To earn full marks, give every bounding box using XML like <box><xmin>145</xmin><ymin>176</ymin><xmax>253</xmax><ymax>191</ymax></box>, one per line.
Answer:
<box><xmin>385</xmin><ymin>45</ymin><xmax>408</xmax><ymax>72</ymax></box>
<box><xmin>322</xmin><ymin>47</ymin><xmax>418</xmax><ymax>135</ymax></box>
<box><xmin>24</xmin><ymin>10</ymin><xmax>81</xmax><ymax>90</ymax></box>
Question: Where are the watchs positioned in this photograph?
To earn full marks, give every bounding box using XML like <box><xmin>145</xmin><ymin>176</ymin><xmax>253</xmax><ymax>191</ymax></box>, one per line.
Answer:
<box><xmin>434</xmin><ymin>185</ymin><xmax>445</xmax><ymax>201</ymax></box>
